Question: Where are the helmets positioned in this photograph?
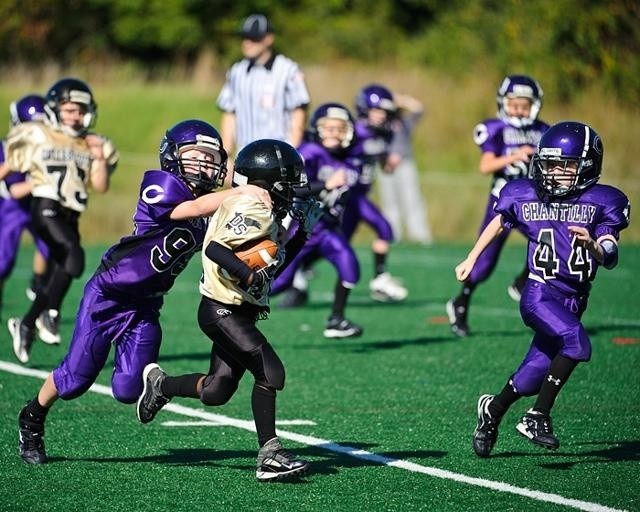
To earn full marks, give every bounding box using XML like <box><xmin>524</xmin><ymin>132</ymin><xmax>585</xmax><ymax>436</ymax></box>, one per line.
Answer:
<box><xmin>8</xmin><ymin>94</ymin><xmax>48</xmax><ymax>127</ymax></box>
<box><xmin>42</xmin><ymin>77</ymin><xmax>98</xmax><ymax>138</ymax></box>
<box><xmin>355</xmin><ymin>82</ymin><xmax>397</xmax><ymax>132</ymax></box>
<box><xmin>496</xmin><ymin>73</ymin><xmax>544</xmax><ymax>129</ymax></box>
<box><xmin>310</xmin><ymin>102</ymin><xmax>358</xmax><ymax>156</ymax></box>
<box><xmin>231</xmin><ymin>137</ymin><xmax>310</xmax><ymax>219</ymax></box>
<box><xmin>537</xmin><ymin>121</ymin><xmax>605</xmax><ymax>198</ymax></box>
<box><xmin>158</xmin><ymin>117</ymin><xmax>229</xmax><ymax>186</ymax></box>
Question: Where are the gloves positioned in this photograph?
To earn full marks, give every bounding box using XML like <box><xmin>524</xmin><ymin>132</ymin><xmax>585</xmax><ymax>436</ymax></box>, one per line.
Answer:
<box><xmin>283</xmin><ymin>199</ymin><xmax>327</xmax><ymax>256</ymax></box>
<box><xmin>250</xmin><ymin>260</ymin><xmax>282</xmax><ymax>290</ymax></box>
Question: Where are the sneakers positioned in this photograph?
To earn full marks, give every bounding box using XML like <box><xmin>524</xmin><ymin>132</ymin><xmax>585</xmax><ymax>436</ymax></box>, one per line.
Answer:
<box><xmin>16</xmin><ymin>404</ymin><xmax>49</xmax><ymax>465</ymax></box>
<box><xmin>255</xmin><ymin>448</ymin><xmax>309</xmax><ymax>481</ymax></box>
<box><xmin>513</xmin><ymin>406</ymin><xmax>561</xmax><ymax>454</ymax></box>
<box><xmin>506</xmin><ymin>275</ymin><xmax>529</xmax><ymax>304</ymax></box>
<box><xmin>367</xmin><ymin>271</ymin><xmax>409</xmax><ymax>305</ymax></box>
<box><xmin>7</xmin><ymin>316</ymin><xmax>36</xmax><ymax>367</ymax></box>
<box><xmin>275</xmin><ymin>269</ymin><xmax>314</xmax><ymax>309</ymax></box>
<box><xmin>135</xmin><ymin>361</ymin><xmax>172</xmax><ymax>424</ymax></box>
<box><xmin>471</xmin><ymin>392</ymin><xmax>502</xmax><ymax>459</ymax></box>
<box><xmin>445</xmin><ymin>294</ymin><xmax>473</xmax><ymax>340</ymax></box>
<box><xmin>321</xmin><ymin>316</ymin><xmax>364</xmax><ymax>340</ymax></box>
<box><xmin>25</xmin><ymin>273</ymin><xmax>65</xmax><ymax>349</ymax></box>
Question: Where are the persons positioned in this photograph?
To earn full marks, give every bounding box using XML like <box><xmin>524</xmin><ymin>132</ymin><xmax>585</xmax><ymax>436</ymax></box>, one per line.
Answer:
<box><xmin>455</xmin><ymin>122</ymin><xmax>631</xmax><ymax>456</ymax></box>
<box><xmin>447</xmin><ymin>75</ymin><xmax>551</xmax><ymax>338</ymax></box>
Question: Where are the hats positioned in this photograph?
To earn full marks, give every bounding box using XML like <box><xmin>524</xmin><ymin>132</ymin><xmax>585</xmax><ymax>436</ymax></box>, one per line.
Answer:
<box><xmin>230</xmin><ymin>12</ymin><xmax>275</xmax><ymax>40</ymax></box>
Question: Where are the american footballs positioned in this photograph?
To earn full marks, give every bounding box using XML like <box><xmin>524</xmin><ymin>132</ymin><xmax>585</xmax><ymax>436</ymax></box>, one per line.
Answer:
<box><xmin>219</xmin><ymin>239</ymin><xmax>277</xmax><ymax>281</ymax></box>
